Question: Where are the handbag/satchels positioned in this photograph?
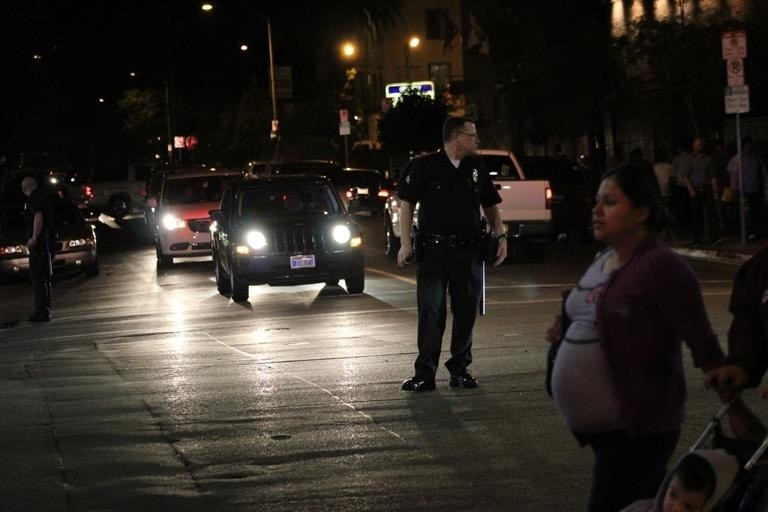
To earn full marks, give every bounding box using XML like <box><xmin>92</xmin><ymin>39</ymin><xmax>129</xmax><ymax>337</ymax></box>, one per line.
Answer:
<box><xmin>544</xmin><ymin>293</ymin><xmax>579</xmax><ymax>400</ymax></box>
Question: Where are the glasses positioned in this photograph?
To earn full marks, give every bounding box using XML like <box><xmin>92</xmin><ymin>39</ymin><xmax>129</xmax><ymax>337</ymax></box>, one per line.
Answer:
<box><xmin>459</xmin><ymin>132</ymin><xmax>478</xmax><ymax>136</ymax></box>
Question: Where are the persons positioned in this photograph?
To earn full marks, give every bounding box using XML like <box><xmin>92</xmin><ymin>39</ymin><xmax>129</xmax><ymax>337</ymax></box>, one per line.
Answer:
<box><xmin>630</xmin><ymin>135</ymin><xmax>768</xmax><ymax>240</ymax></box>
<box><xmin>619</xmin><ymin>447</ymin><xmax>738</xmax><ymax>512</ymax></box>
<box><xmin>702</xmin><ymin>243</ymin><xmax>767</xmax><ymax>399</ymax></box>
<box><xmin>20</xmin><ymin>175</ymin><xmax>58</xmax><ymax>323</ymax></box>
<box><xmin>544</xmin><ymin>160</ymin><xmax>767</xmax><ymax>512</ymax></box>
<box><xmin>394</xmin><ymin>115</ymin><xmax>511</xmax><ymax>394</ymax></box>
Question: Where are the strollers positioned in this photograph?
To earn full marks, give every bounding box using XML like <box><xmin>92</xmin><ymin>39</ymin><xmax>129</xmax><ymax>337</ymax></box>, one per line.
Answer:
<box><xmin>622</xmin><ymin>398</ymin><xmax>768</xmax><ymax>512</ymax></box>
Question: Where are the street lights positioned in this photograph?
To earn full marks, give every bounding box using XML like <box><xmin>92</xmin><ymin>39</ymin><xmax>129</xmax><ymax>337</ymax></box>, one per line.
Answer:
<box><xmin>130</xmin><ymin>72</ymin><xmax>176</xmax><ymax>168</ymax></box>
<box><xmin>202</xmin><ymin>3</ymin><xmax>281</xmax><ymax>164</ymax></box>
<box><xmin>403</xmin><ymin>37</ymin><xmax>419</xmax><ymax>92</ymax></box>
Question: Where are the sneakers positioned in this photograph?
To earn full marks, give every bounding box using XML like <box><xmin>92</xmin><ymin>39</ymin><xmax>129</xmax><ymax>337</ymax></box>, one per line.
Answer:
<box><xmin>27</xmin><ymin>310</ymin><xmax>53</xmax><ymax>322</ymax></box>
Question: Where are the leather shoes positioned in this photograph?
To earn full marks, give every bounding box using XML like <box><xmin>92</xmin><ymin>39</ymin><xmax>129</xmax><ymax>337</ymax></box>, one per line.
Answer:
<box><xmin>399</xmin><ymin>374</ymin><xmax>437</xmax><ymax>393</ymax></box>
<box><xmin>448</xmin><ymin>371</ymin><xmax>481</xmax><ymax>390</ymax></box>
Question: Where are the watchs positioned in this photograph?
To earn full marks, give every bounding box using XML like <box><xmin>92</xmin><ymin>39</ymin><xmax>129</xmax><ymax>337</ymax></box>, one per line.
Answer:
<box><xmin>497</xmin><ymin>233</ymin><xmax>509</xmax><ymax>240</ymax></box>
